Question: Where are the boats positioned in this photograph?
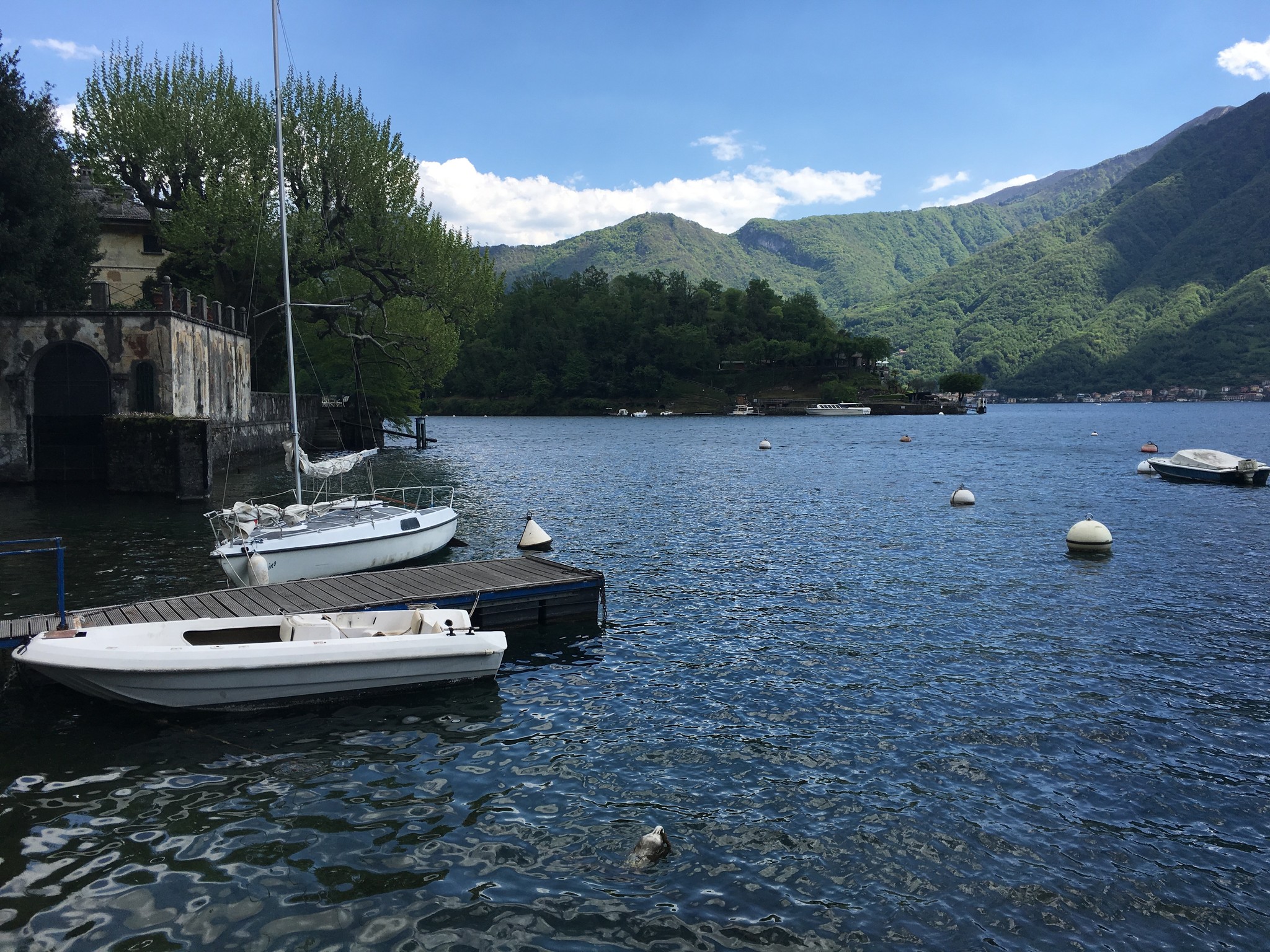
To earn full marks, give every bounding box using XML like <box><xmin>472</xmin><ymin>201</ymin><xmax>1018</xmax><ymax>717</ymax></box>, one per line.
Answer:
<box><xmin>205</xmin><ymin>484</ymin><xmax>461</xmax><ymax>589</ymax></box>
<box><xmin>617</xmin><ymin>409</ymin><xmax>628</xmax><ymax>415</ymax></box>
<box><xmin>631</xmin><ymin>413</ymin><xmax>647</xmax><ymax>417</ymax></box>
<box><xmin>804</xmin><ymin>402</ymin><xmax>871</xmax><ymax>415</ymax></box>
<box><xmin>11</xmin><ymin>605</ymin><xmax>507</xmax><ymax>715</ymax></box>
<box><xmin>733</xmin><ymin>405</ymin><xmax>754</xmax><ymax>415</ymax></box>
<box><xmin>1145</xmin><ymin>450</ymin><xmax>1270</xmax><ymax>488</ymax></box>
